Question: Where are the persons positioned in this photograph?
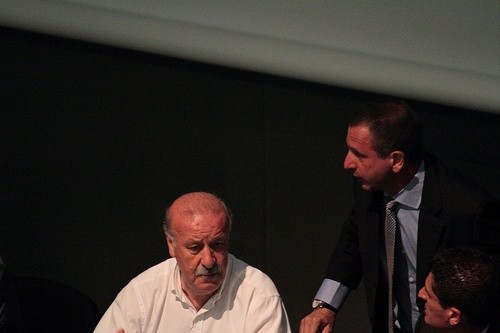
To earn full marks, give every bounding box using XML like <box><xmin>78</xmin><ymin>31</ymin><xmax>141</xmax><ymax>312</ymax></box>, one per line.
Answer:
<box><xmin>297</xmin><ymin>101</ymin><xmax>500</xmax><ymax>333</ymax></box>
<box><xmin>92</xmin><ymin>192</ymin><xmax>293</xmax><ymax>333</ymax></box>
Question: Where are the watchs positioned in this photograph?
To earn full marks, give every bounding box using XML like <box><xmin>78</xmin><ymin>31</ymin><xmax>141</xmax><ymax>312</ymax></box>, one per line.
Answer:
<box><xmin>312</xmin><ymin>300</ymin><xmax>338</xmax><ymax>313</ymax></box>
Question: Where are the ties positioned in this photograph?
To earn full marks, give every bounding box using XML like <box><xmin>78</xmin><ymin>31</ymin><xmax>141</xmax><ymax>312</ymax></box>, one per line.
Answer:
<box><xmin>384</xmin><ymin>197</ymin><xmax>397</xmax><ymax>333</ymax></box>
